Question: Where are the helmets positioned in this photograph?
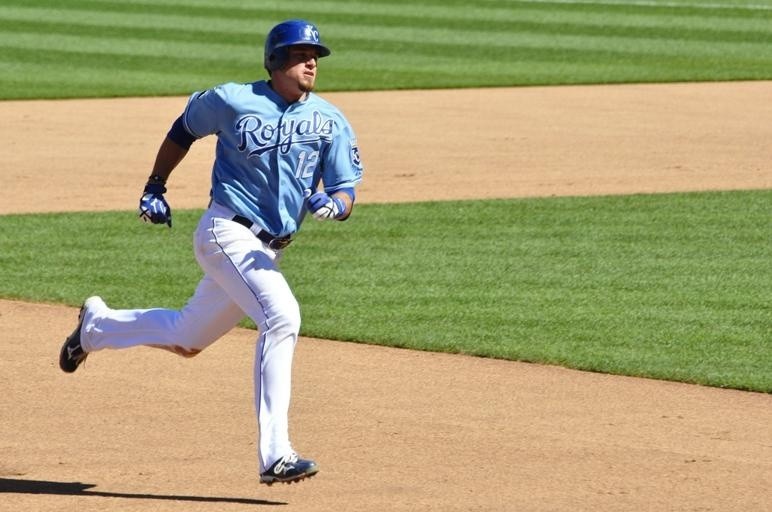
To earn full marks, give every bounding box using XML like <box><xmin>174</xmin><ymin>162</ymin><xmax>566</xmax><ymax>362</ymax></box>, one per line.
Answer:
<box><xmin>264</xmin><ymin>20</ymin><xmax>331</xmax><ymax>67</ymax></box>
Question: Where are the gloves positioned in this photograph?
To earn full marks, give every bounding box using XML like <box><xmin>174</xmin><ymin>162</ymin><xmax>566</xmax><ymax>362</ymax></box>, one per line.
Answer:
<box><xmin>140</xmin><ymin>184</ymin><xmax>172</xmax><ymax>227</ymax></box>
<box><xmin>306</xmin><ymin>192</ymin><xmax>346</xmax><ymax>221</ymax></box>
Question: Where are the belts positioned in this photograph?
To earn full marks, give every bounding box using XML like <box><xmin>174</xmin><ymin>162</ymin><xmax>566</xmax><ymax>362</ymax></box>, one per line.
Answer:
<box><xmin>232</xmin><ymin>214</ymin><xmax>293</xmax><ymax>249</ymax></box>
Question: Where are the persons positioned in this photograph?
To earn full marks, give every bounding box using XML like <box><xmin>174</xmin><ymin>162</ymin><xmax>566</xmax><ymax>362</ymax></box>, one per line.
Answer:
<box><xmin>58</xmin><ymin>18</ymin><xmax>363</xmax><ymax>485</ymax></box>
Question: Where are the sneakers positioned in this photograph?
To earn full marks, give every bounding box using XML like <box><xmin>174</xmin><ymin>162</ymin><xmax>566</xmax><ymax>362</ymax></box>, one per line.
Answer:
<box><xmin>260</xmin><ymin>451</ymin><xmax>320</xmax><ymax>487</ymax></box>
<box><xmin>58</xmin><ymin>296</ymin><xmax>102</xmax><ymax>374</ymax></box>
